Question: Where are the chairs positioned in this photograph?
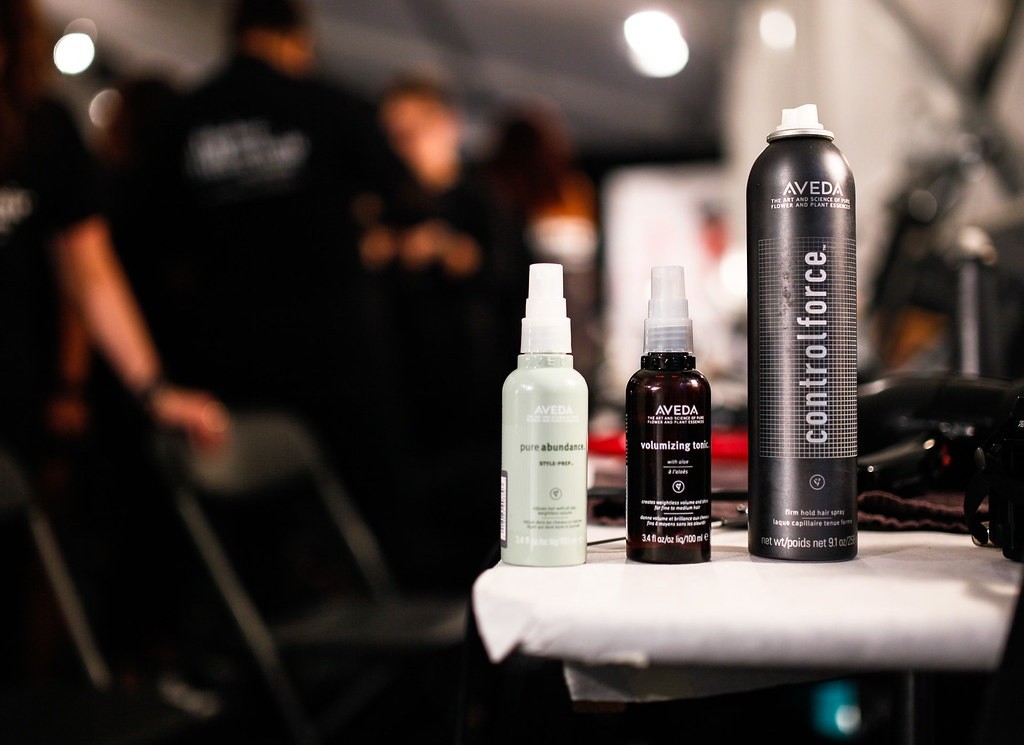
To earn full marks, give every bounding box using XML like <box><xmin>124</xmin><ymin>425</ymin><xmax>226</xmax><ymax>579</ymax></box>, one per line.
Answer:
<box><xmin>170</xmin><ymin>415</ymin><xmax>472</xmax><ymax>745</ymax></box>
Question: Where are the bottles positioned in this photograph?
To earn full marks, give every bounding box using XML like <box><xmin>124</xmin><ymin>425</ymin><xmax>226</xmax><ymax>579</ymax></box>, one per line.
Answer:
<box><xmin>745</xmin><ymin>103</ymin><xmax>857</xmax><ymax>563</ymax></box>
<box><xmin>625</xmin><ymin>266</ymin><xmax>711</xmax><ymax>564</ymax></box>
<box><xmin>499</xmin><ymin>262</ymin><xmax>588</xmax><ymax>567</ymax></box>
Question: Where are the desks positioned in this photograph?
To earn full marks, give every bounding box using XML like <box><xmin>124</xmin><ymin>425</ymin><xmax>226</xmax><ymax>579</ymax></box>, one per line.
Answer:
<box><xmin>467</xmin><ymin>507</ymin><xmax>1024</xmax><ymax>745</ymax></box>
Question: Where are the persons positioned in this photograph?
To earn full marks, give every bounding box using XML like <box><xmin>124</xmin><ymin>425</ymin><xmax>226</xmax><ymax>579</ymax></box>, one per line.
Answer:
<box><xmin>671</xmin><ymin>204</ymin><xmax>748</xmax><ymax>381</ymax></box>
<box><xmin>0</xmin><ymin>0</ymin><xmax>594</xmax><ymax>716</ymax></box>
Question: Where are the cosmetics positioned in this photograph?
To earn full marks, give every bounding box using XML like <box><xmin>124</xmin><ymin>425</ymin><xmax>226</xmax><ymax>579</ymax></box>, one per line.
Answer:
<box><xmin>501</xmin><ymin>263</ymin><xmax>588</xmax><ymax>567</ymax></box>
<box><xmin>628</xmin><ymin>267</ymin><xmax>712</xmax><ymax>564</ymax></box>
